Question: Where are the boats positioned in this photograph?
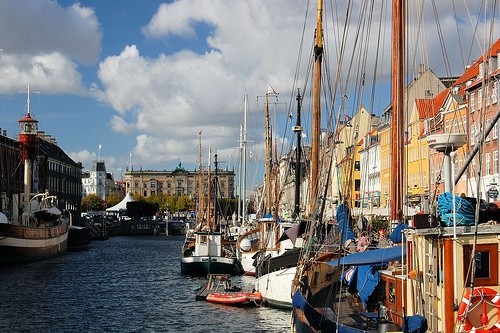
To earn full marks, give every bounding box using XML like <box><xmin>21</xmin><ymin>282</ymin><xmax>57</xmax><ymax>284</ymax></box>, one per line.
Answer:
<box><xmin>194</xmin><ymin>273</ymin><xmax>262</xmax><ymax>305</ymax></box>
<box><xmin>0</xmin><ymin>85</ymin><xmax>71</xmax><ymax>258</ymax></box>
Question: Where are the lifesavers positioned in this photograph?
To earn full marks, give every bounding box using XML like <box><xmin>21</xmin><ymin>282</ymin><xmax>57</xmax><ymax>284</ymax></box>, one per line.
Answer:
<box><xmin>457</xmin><ymin>288</ymin><xmax>500</xmax><ymax>333</ymax></box>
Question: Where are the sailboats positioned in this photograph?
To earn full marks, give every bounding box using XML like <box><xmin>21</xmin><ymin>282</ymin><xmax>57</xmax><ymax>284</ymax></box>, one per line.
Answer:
<box><xmin>178</xmin><ymin>0</ymin><xmax>500</xmax><ymax>333</ymax></box>
